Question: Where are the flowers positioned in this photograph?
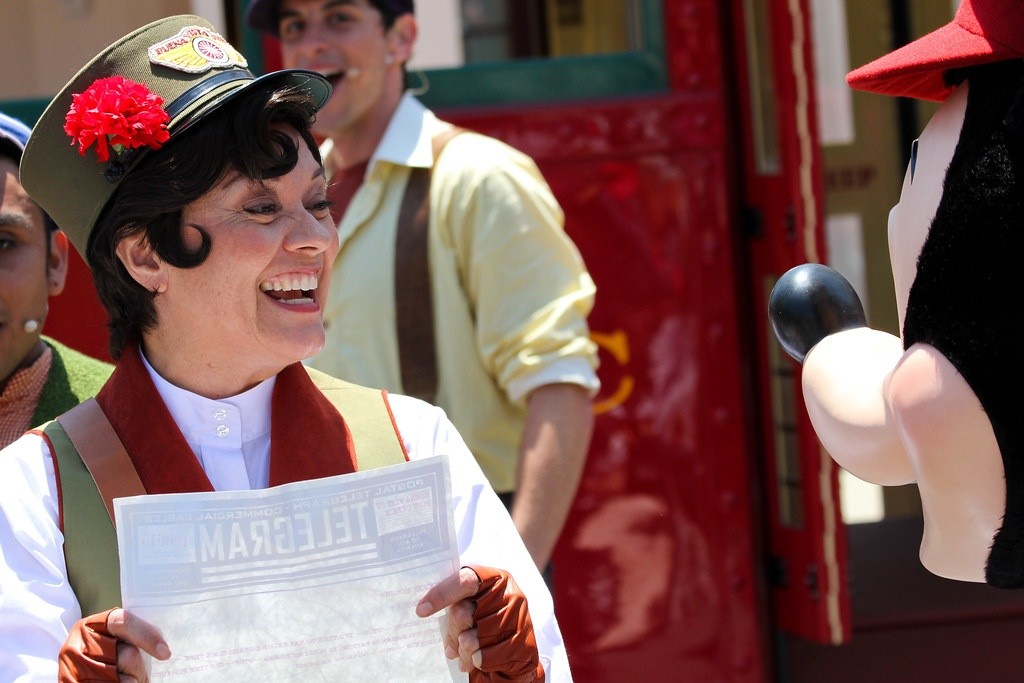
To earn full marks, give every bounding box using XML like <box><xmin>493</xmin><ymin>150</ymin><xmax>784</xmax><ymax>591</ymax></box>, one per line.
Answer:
<box><xmin>63</xmin><ymin>74</ymin><xmax>172</xmax><ymax>162</ymax></box>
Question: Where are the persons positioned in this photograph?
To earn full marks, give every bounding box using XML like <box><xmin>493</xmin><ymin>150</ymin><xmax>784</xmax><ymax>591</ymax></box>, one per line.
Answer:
<box><xmin>247</xmin><ymin>0</ymin><xmax>601</xmax><ymax>597</ymax></box>
<box><xmin>0</xmin><ymin>15</ymin><xmax>574</xmax><ymax>683</ymax></box>
<box><xmin>0</xmin><ymin>111</ymin><xmax>116</xmax><ymax>451</ymax></box>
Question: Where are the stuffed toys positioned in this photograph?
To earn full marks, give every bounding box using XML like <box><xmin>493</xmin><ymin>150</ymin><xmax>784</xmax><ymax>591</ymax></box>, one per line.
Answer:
<box><xmin>768</xmin><ymin>0</ymin><xmax>1024</xmax><ymax>589</ymax></box>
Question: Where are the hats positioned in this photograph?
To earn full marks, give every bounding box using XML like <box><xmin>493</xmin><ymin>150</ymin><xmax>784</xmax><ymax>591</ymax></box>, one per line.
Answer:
<box><xmin>247</xmin><ymin>0</ymin><xmax>287</xmax><ymax>37</ymax></box>
<box><xmin>18</xmin><ymin>15</ymin><xmax>334</xmax><ymax>273</ymax></box>
<box><xmin>0</xmin><ymin>113</ymin><xmax>33</xmax><ymax>152</ymax></box>
